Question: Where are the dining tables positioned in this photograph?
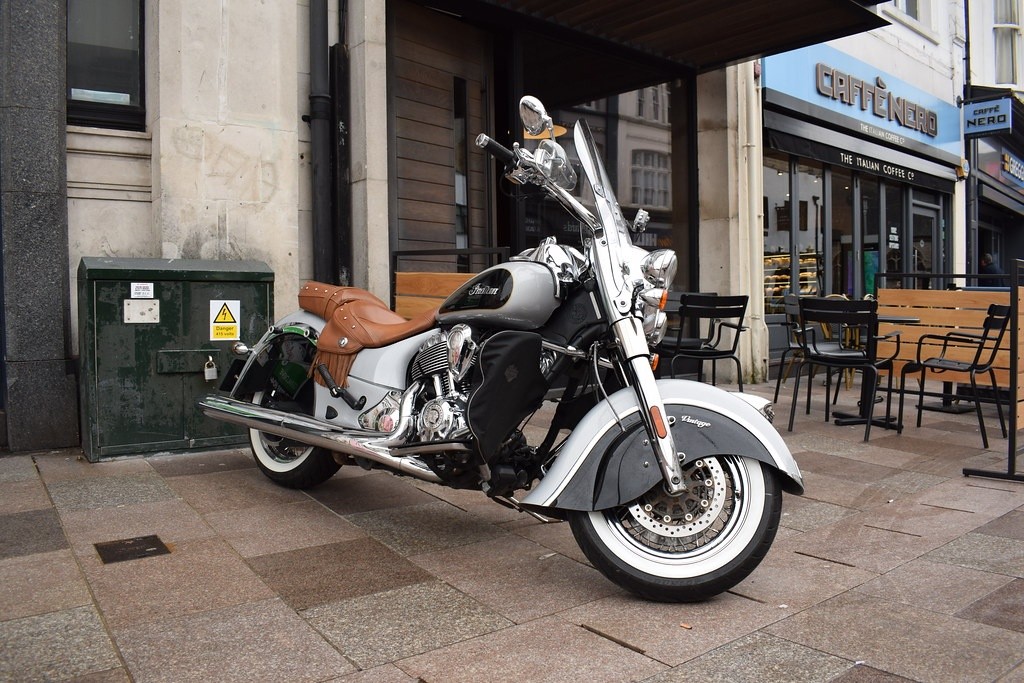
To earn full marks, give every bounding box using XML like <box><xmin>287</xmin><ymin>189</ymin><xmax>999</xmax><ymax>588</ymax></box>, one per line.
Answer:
<box><xmin>878</xmin><ymin>315</ymin><xmax>921</xmax><ymax>323</ymax></box>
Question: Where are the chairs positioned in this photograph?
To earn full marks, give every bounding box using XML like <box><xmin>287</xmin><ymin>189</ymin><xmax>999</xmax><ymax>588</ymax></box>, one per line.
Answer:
<box><xmin>812</xmin><ymin>294</ymin><xmax>855</xmax><ymax>389</ymax></box>
<box><xmin>788</xmin><ymin>297</ymin><xmax>902</xmax><ymax>442</ymax></box>
<box><xmin>897</xmin><ymin>304</ymin><xmax>1011</xmax><ymax>448</ymax></box>
<box><xmin>662</xmin><ymin>292</ymin><xmax>718</xmax><ymax>382</ymax></box>
<box><xmin>773</xmin><ymin>296</ymin><xmax>841</xmax><ymax>414</ymax></box>
<box><xmin>658</xmin><ymin>293</ymin><xmax>749</xmax><ymax>393</ymax></box>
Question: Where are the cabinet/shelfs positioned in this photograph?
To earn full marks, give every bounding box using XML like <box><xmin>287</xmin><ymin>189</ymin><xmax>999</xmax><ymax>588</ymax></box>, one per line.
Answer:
<box><xmin>764</xmin><ymin>253</ymin><xmax>824</xmax><ymax>314</ymax></box>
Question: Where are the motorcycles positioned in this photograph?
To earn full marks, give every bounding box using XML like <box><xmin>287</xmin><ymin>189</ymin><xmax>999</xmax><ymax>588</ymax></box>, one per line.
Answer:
<box><xmin>197</xmin><ymin>94</ymin><xmax>805</xmax><ymax>605</ymax></box>
